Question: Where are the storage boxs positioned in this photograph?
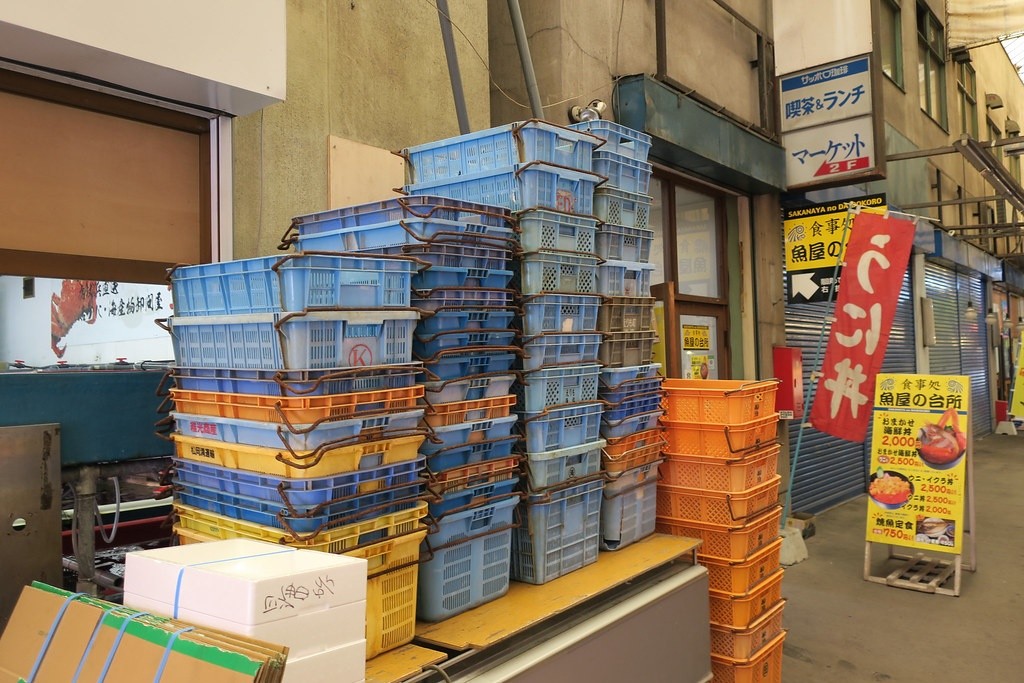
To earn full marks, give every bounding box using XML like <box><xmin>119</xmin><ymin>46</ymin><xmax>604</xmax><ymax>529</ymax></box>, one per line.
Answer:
<box><xmin>124</xmin><ymin>118</ymin><xmax>786</xmax><ymax>683</ymax></box>
